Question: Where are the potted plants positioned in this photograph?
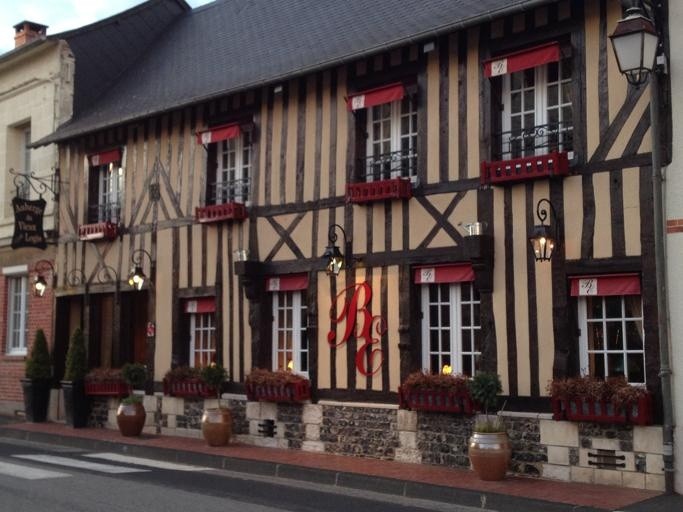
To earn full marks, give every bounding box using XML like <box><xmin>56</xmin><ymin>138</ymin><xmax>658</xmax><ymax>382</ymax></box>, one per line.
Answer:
<box><xmin>468</xmin><ymin>371</ymin><xmax>511</xmax><ymax>481</ymax></box>
<box><xmin>545</xmin><ymin>377</ymin><xmax>653</xmax><ymax>424</ymax></box>
<box><xmin>84</xmin><ymin>368</ymin><xmax>133</xmax><ymax>395</ymax></box>
<box><xmin>200</xmin><ymin>364</ymin><xmax>233</xmax><ymax>447</ymax></box>
<box><xmin>403</xmin><ymin>370</ymin><xmax>482</xmax><ymax>414</ymax></box>
<box><xmin>165</xmin><ymin>366</ymin><xmax>217</xmax><ymax>396</ymax></box>
<box><xmin>116</xmin><ymin>360</ymin><xmax>151</xmax><ymax>436</ymax></box>
<box><xmin>245</xmin><ymin>368</ymin><xmax>311</xmax><ymax>401</ymax></box>
<box><xmin>19</xmin><ymin>329</ymin><xmax>51</xmax><ymax>423</ymax></box>
<box><xmin>60</xmin><ymin>328</ymin><xmax>91</xmax><ymax>429</ymax></box>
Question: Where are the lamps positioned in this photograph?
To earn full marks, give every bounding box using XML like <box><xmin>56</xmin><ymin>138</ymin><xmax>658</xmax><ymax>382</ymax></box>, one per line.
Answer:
<box><xmin>321</xmin><ymin>224</ymin><xmax>352</xmax><ymax>275</ymax></box>
<box><xmin>127</xmin><ymin>249</ymin><xmax>156</xmax><ymax>290</ymax></box>
<box><xmin>31</xmin><ymin>259</ymin><xmax>57</xmax><ymax>297</ymax></box>
<box><xmin>608</xmin><ymin>0</ymin><xmax>668</xmax><ymax>90</ymax></box>
<box><xmin>528</xmin><ymin>198</ymin><xmax>563</xmax><ymax>262</ymax></box>
